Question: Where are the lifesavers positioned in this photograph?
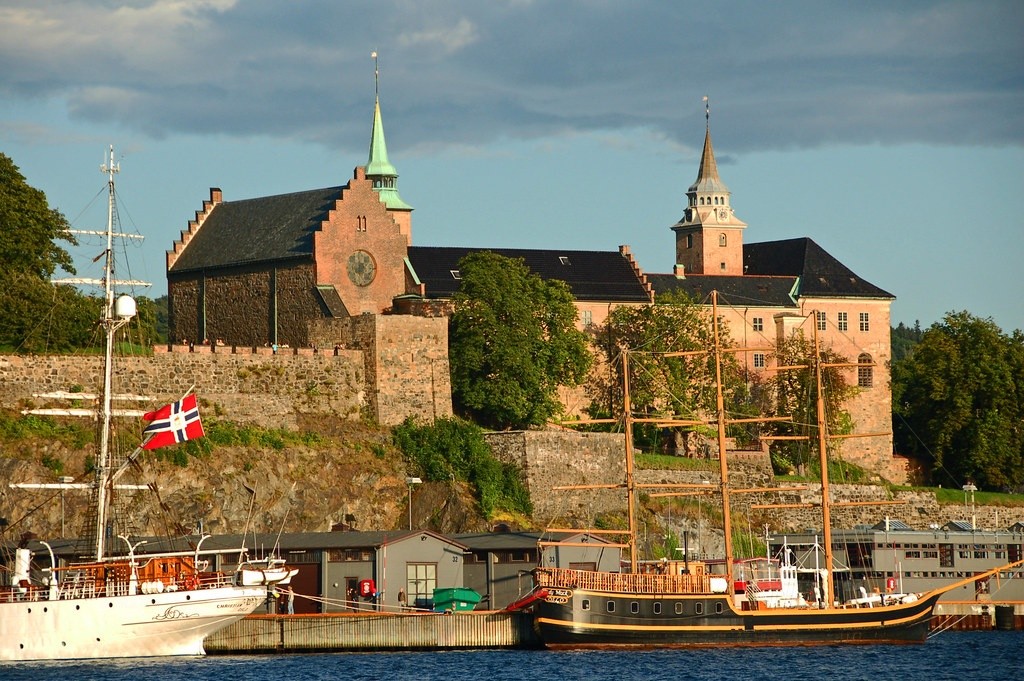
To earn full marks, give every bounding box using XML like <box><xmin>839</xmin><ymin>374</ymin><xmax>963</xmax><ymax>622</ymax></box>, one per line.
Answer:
<box><xmin>184</xmin><ymin>575</ymin><xmax>198</xmax><ymax>590</ymax></box>
<box><xmin>888</xmin><ymin>577</ymin><xmax>896</xmax><ymax>589</ymax></box>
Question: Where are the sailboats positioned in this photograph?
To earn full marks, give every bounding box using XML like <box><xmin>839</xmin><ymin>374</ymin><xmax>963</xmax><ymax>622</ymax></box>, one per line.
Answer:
<box><xmin>531</xmin><ymin>288</ymin><xmax>1023</xmax><ymax>653</ymax></box>
<box><xmin>0</xmin><ymin>143</ymin><xmax>300</xmax><ymax>663</ymax></box>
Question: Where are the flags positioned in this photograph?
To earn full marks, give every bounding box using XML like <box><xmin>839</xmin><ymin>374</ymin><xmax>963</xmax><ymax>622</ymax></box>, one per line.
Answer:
<box><xmin>140</xmin><ymin>391</ymin><xmax>206</xmax><ymax>451</ymax></box>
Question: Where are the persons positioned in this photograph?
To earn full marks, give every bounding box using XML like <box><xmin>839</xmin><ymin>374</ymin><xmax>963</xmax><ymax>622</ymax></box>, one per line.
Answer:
<box><xmin>180</xmin><ymin>337</ymin><xmax>346</xmax><ymax>356</ymax></box>
<box><xmin>398</xmin><ymin>587</ymin><xmax>406</xmax><ymax>612</ymax></box>
<box><xmin>288</xmin><ymin>586</ymin><xmax>294</xmax><ymax>614</ymax></box>
<box><xmin>368</xmin><ymin>593</ymin><xmax>377</xmax><ymax>610</ymax></box>
<box><xmin>873</xmin><ymin>585</ymin><xmax>903</xmax><ymax>606</ymax></box>
<box><xmin>352</xmin><ymin>589</ymin><xmax>359</xmax><ymax>613</ymax></box>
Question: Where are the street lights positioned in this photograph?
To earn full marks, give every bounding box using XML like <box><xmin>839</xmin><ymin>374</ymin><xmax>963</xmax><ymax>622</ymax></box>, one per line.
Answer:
<box><xmin>405</xmin><ymin>475</ymin><xmax>423</xmax><ymax>531</ymax></box>
